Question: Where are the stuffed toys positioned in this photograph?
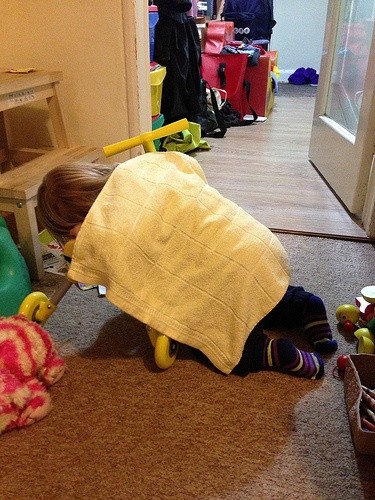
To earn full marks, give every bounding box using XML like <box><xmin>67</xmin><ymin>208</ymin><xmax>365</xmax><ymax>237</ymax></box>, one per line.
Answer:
<box><xmin>0</xmin><ymin>314</ymin><xmax>65</xmax><ymax>434</ymax></box>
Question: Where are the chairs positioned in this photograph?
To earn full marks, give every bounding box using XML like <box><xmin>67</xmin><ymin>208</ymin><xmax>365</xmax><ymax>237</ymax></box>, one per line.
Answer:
<box><xmin>204</xmin><ymin>21</ymin><xmax>278</xmax><ymax>117</ymax></box>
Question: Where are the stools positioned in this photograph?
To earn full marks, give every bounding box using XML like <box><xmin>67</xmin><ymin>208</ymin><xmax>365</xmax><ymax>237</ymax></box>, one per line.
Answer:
<box><xmin>0</xmin><ymin>145</ymin><xmax>103</xmax><ymax>282</ymax></box>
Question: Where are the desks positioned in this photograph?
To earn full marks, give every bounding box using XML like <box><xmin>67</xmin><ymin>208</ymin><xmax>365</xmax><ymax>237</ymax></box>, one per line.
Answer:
<box><xmin>1</xmin><ymin>70</ymin><xmax>69</xmax><ymax>171</ymax></box>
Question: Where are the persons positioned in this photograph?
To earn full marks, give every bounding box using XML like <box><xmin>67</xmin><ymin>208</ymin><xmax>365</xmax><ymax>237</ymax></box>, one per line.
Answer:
<box><xmin>36</xmin><ymin>151</ymin><xmax>339</xmax><ymax>380</ymax></box>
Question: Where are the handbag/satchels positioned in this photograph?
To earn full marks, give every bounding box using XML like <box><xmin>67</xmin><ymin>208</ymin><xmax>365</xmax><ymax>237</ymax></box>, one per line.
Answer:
<box><xmin>201</xmin><ymin>44</ymin><xmax>279</xmax><ymax>122</ymax></box>
